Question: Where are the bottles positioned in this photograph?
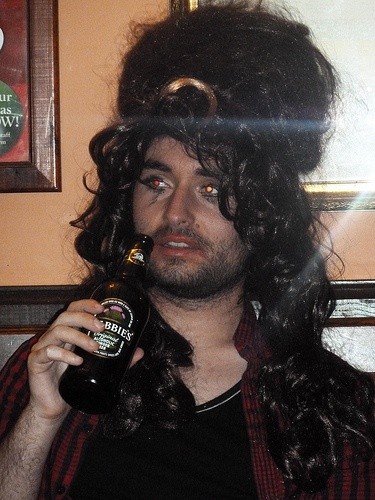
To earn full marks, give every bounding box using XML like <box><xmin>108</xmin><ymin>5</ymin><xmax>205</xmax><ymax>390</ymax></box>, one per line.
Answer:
<box><xmin>58</xmin><ymin>232</ymin><xmax>159</xmax><ymax>416</ymax></box>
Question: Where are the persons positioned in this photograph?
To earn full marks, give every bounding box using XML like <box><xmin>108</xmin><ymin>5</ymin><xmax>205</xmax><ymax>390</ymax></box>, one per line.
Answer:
<box><xmin>0</xmin><ymin>1</ymin><xmax>375</xmax><ymax>500</ymax></box>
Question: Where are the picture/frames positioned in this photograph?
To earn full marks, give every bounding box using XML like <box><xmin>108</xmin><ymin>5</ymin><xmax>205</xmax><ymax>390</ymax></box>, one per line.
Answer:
<box><xmin>170</xmin><ymin>0</ymin><xmax>374</xmax><ymax>211</ymax></box>
<box><xmin>0</xmin><ymin>0</ymin><xmax>65</xmax><ymax>192</ymax></box>
